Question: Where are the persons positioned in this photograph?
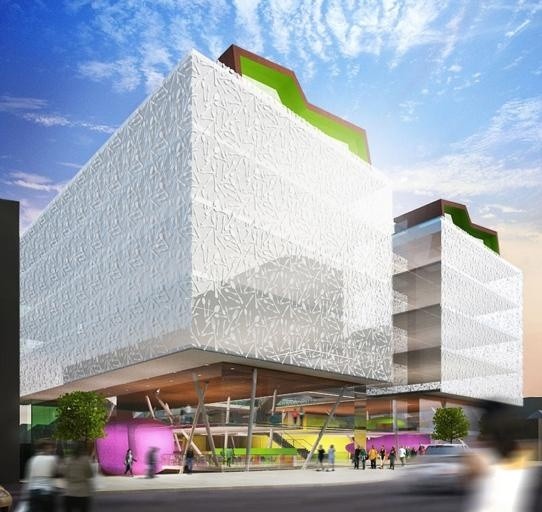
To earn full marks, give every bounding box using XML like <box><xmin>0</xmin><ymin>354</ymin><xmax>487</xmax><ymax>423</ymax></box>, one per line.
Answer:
<box><xmin>225</xmin><ymin>445</ymin><xmax>234</xmax><ymax>467</ymax></box>
<box><xmin>186</xmin><ymin>446</ymin><xmax>194</xmax><ymax>472</ymax></box>
<box><xmin>298</xmin><ymin>406</ymin><xmax>305</xmax><ymax>428</ymax></box>
<box><xmin>122</xmin><ymin>448</ymin><xmax>139</xmax><ymax>478</ymax></box>
<box><xmin>292</xmin><ymin>408</ymin><xmax>299</xmax><ymax>426</ymax></box>
<box><xmin>178</xmin><ymin>404</ymin><xmax>186</xmax><ymax>424</ymax></box>
<box><xmin>144</xmin><ymin>446</ymin><xmax>160</xmax><ymax>480</ymax></box>
<box><xmin>456</xmin><ymin>406</ymin><xmax>542</xmax><ymax>510</ymax></box>
<box><xmin>24</xmin><ymin>437</ymin><xmax>64</xmax><ymax>511</ymax></box>
<box><xmin>60</xmin><ymin>440</ymin><xmax>97</xmax><ymax>511</ymax></box>
<box><xmin>316</xmin><ymin>444</ymin><xmax>426</xmax><ymax>474</ymax></box>
